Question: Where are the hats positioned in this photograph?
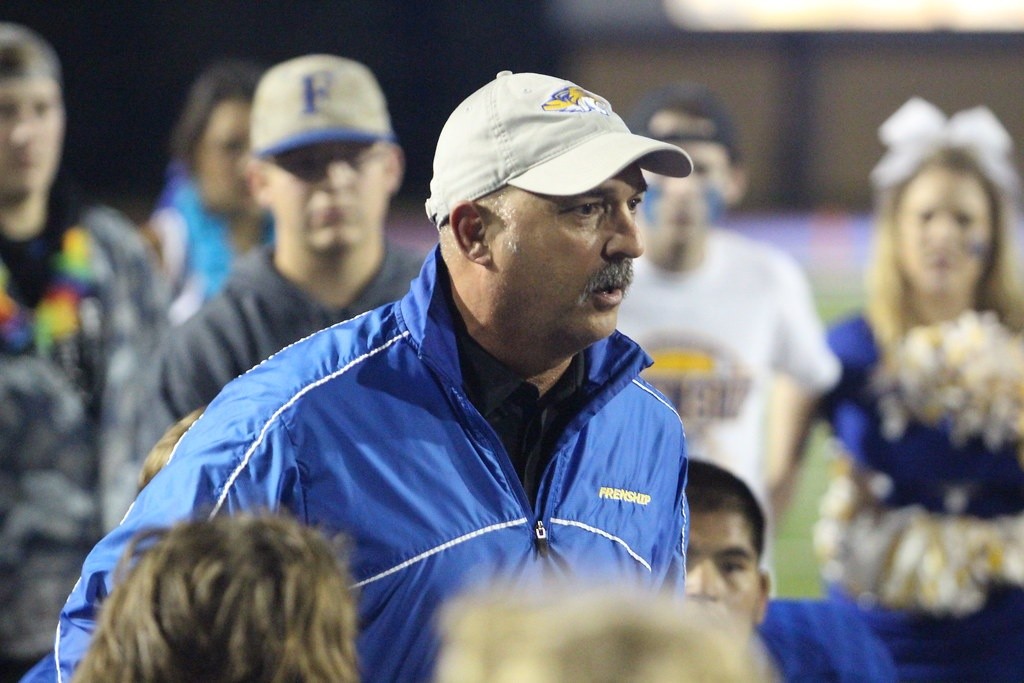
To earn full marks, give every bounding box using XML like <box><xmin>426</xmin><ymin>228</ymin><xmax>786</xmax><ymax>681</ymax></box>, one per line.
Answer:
<box><xmin>242</xmin><ymin>52</ymin><xmax>397</xmax><ymax>161</ymax></box>
<box><xmin>424</xmin><ymin>68</ymin><xmax>693</xmax><ymax>231</ymax></box>
<box><xmin>634</xmin><ymin>78</ymin><xmax>740</xmax><ymax>164</ymax></box>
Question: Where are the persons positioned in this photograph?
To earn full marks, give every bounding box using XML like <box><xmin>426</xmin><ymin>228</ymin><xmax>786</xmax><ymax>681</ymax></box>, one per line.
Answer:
<box><xmin>53</xmin><ymin>68</ymin><xmax>692</xmax><ymax>683</ymax></box>
<box><xmin>125</xmin><ymin>53</ymin><xmax>424</xmax><ymax>480</ymax></box>
<box><xmin>825</xmin><ymin>101</ymin><xmax>1024</xmax><ymax>683</ymax></box>
<box><xmin>432</xmin><ymin>578</ymin><xmax>782</xmax><ymax>682</ymax></box>
<box><xmin>143</xmin><ymin>61</ymin><xmax>275</xmax><ymax>327</ymax></box>
<box><xmin>75</xmin><ymin>512</ymin><xmax>360</xmax><ymax>683</ymax></box>
<box><xmin>0</xmin><ymin>24</ymin><xmax>171</xmax><ymax>683</ymax></box>
<box><xmin>680</xmin><ymin>460</ymin><xmax>772</xmax><ymax>683</ymax></box>
<box><xmin>616</xmin><ymin>99</ymin><xmax>843</xmax><ymax>493</ymax></box>
